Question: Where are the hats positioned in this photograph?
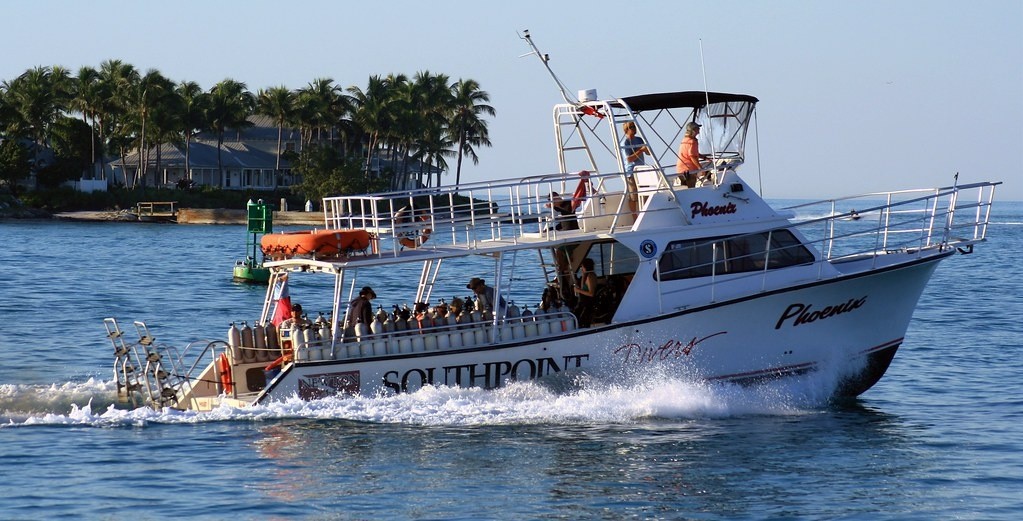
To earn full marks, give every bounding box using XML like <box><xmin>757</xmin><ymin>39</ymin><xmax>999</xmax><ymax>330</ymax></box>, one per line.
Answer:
<box><xmin>360</xmin><ymin>287</ymin><xmax>377</xmax><ymax>299</ymax></box>
<box><xmin>291</xmin><ymin>304</ymin><xmax>302</xmax><ymax>310</ymax></box>
<box><xmin>687</xmin><ymin>122</ymin><xmax>702</xmax><ymax>130</ymax></box>
<box><xmin>466</xmin><ymin>278</ymin><xmax>484</xmax><ymax>288</ymax></box>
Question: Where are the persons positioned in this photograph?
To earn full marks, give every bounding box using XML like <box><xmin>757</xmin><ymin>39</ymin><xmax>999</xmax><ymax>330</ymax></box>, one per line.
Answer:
<box><xmin>544</xmin><ymin>191</ymin><xmax>580</xmax><ymax>232</ymax></box>
<box><xmin>619</xmin><ymin>120</ymin><xmax>650</xmax><ymax>221</ymax></box>
<box><xmin>269</xmin><ymin>271</ymin><xmax>292</xmax><ymax>324</ymax></box>
<box><xmin>675</xmin><ymin>122</ymin><xmax>710</xmax><ymax>188</ymax></box>
<box><xmin>465</xmin><ymin>278</ymin><xmax>512</xmax><ymax>318</ymax></box>
<box><xmin>283</xmin><ymin>303</ymin><xmax>313</xmax><ymax>342</ymax></box>
<box><xmin>571</xmin><ymin>257</ymin><xmax>598</xmax><ymax>328</ymax></box>
<box><xmin>348</xmin><ymin>286</ymin><xmax>377</xmax><ymax>339</ymax></box>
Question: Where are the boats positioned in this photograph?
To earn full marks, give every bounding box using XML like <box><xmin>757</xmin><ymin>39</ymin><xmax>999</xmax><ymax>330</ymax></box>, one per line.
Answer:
<box><xmin>100</xmin><ymin>27</ymin><xmax>1004</xmax><ymax>414</ymax></box>
<box><xmin>260</xmin><ymin>228</ymin><xmax>370</xmax><ymax>261</ymax></box>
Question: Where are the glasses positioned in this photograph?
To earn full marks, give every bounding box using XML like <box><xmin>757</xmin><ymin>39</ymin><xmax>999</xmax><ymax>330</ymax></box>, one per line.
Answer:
<box><xmin>629</xmin><ymin>126</ymin><xmax>635</xmax><ymax>129</ymax></box>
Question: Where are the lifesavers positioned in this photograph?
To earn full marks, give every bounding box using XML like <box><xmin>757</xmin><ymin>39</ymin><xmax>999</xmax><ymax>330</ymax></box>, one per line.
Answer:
<box><xmin>218</xmin><ymin>353</ymin><xmax>233</xmax><ymax>396</ymax></box>
<box><xmin>391</xmin><ymin>205</ymin><xmax>433</xmax><ymax>248</ymax></box>
